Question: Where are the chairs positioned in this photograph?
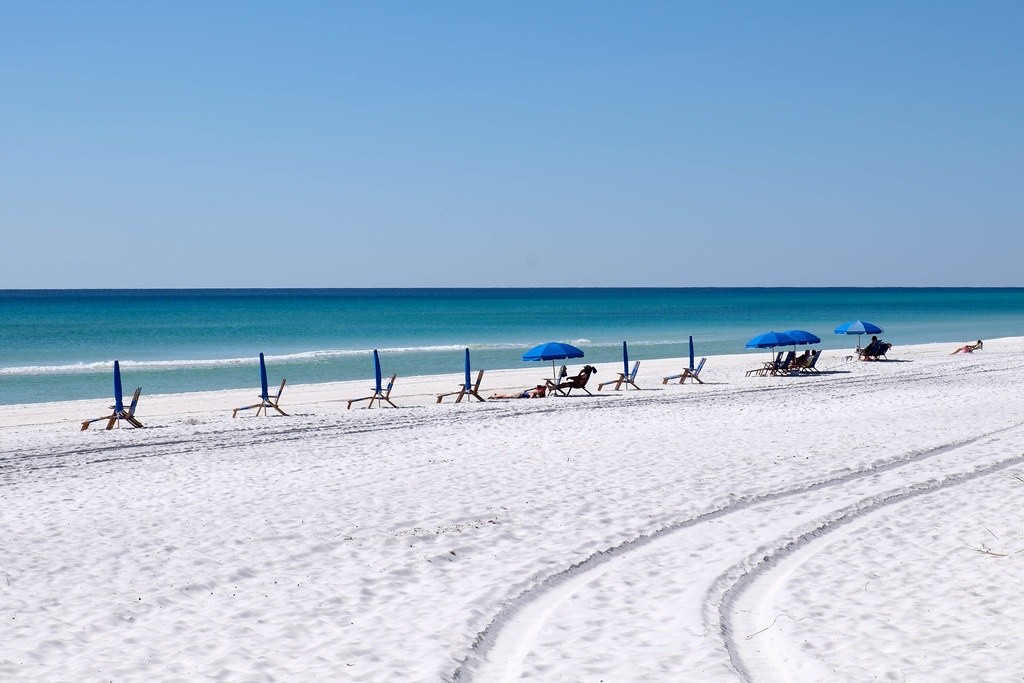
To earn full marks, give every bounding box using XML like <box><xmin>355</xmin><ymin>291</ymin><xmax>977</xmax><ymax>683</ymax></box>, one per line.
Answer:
<box><xmin>745</xmin><ymin>349</ymin><xmax>822</xmax><ymax>377</ymax></box>
<box><xmin>438</xmin><ymin>368</ymin><xmax>484</xmax><ymax>403</ymax></box>
<box><xmin>857</xmin><ymin>339</ymin><xmax>891</xmax><ymax>361</ymax></box>
<box><xmin>663</xmin><ymin>358</ymin><xmax>706</xmax><ymax>385</ymax></box>
<box><xmin>963</xmin><ymin>343</ymin><xmax>983</xmax><ymax>353</ymax></box>
<box><xmin>81</xmin><ymin>387</ymin><xmax>143</xmax><ymax>431</ymax></box>
<box><xmin>566</xmin><ymin>367</ymin><xmax>594</xmax><ymax>396</ymax></box>
<box><xmin>346</xmin><ymin>373</ymin><xmax>397</xmax><ymax>410</ymax></box>
<box><xmin>545</xmin><ymin>366</ymin><xmax>566</xmax><ymax>396</ymax></box>
<box><xmin>233</xmin><ymin>380</ymin><xmax>289</xmax><ymax>418</ymax></box>
<box><xmin>598</xmin><ymin>361</ymin><xmax>642</xmax><ymax>391</ymax></box>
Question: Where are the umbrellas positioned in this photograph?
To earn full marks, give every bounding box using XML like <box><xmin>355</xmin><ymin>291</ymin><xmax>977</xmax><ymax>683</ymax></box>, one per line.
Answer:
<box><xmin>114</xmin><ymin>360</ymin><xmax>123</xmax><ymax>428</ymax></box>
<box><xmin>374</xmin><ymin>350</ymin><xmax>381</xmax><ymax>407</ymax></box>
<box><xmin>623</xmin><ymin>340</ymin><xmax>628</xmax><ymax>390</ymax></box>
<box><xmin>689</xmin><ymin>334</ymin><xmax>694</xmax><ymax>384</ymax></box>
<box><xmin>834</xmin><ymin>320</ymin><xmax>884</xmax><ymax>359</ymax></box>
<box><xmin>260</xmin><ymin>353</ymin><xmax>268</xmax><ymax>416</ymax></box>
<box><xmin>522</xmin><ymin>342</ymin><xmax>584</xmax><ymax>393</ymax></box>
<box><xmin>745</xmin><ymin>329</ymin><xmax>820</xmax><ymax>365</ymax></box>
<box><xmin>465</xmin><ymin>348</ymin><xmax>471</xmax><ymax>401</ymax></box>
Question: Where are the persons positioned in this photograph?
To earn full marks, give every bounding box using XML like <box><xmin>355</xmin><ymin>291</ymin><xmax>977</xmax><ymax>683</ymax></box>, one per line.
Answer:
<box><xmin>950</xmin><ymin>340</ymin><xmax>983</xmax><ymax>355</ymax></box>
<box><xmin>488</xmin><ymin>384</ymin><xmax>547</xmax><ymax>399</ymax></box>
<box><xmin>792</xmin><ymin>350</ymin><xmax>816</xmax><ymax>368</ymax></box>
<box><xmin>863</xmin><ymin>336</ymin><xmax>877</xmax><ymax>361</ymax></box>
<box><xmin>545</xmin><ymin>365</ymin><xmax>597</xmax><ymax>390</ymax></box>
